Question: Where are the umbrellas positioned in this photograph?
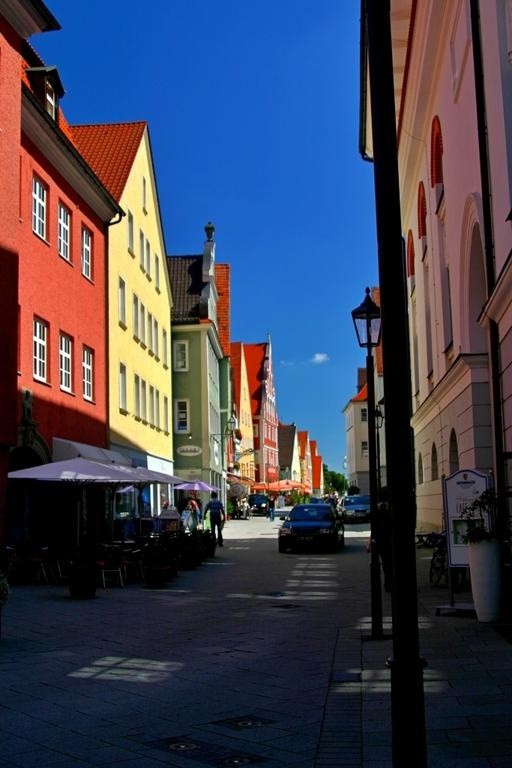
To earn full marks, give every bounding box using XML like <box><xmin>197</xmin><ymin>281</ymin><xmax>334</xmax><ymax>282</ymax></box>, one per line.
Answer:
<box><xmin>6</xmin><ymin>454</ymin><xmax>144</xmax><ymax>550</ymax></box>
<box><xmin>116</xmin><ymin>465</ymin><xmax>227</xmax><ymax>548</ymax></box>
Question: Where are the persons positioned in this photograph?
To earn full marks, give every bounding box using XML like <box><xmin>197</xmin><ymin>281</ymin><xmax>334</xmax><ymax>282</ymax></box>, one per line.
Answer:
<box><xmin>234</xmin><ymin>477</ymin><xmax>339</xmax><ymax>522</ymax></box>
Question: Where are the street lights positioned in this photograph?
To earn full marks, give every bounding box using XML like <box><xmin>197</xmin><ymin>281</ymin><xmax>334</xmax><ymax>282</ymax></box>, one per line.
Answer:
<box><xmin>372</xmin><ymin>404</ymin><xmax>383</xmax><ymax>498</ymax></box>
<box><xmin>352</xmin><ymin>286</ymin><xmax>391</xmax><ymax>643</ymax></box>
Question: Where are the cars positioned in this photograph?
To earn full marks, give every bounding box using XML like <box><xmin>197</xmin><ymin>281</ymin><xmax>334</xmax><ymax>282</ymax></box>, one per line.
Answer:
<box><xmin>338</xmin><ymin>495</ymin><xmax>370</xmax><ymax>520</ymax></box>
<box><xmin>276</xmin><ymin>505</ymin><xmax>346</xmax><ymax>550</ymax></box>
<box><xmin>248</xmin><ymin>493</ymin><xmax>271</xmax><ymax>518</ymax></box>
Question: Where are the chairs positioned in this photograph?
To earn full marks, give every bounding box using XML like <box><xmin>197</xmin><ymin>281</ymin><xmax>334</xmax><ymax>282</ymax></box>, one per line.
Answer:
<box><xmin>36</xmin><ymin>530</ymin><xmax>219</xmax><ymax>590</ymax></box>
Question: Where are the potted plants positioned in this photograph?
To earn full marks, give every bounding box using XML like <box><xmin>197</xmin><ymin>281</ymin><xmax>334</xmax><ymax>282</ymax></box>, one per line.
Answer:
<box><xmin>463</xmin><ymin>484</ymin><xmax>503</xmax><ymax>622</ymax></box>
